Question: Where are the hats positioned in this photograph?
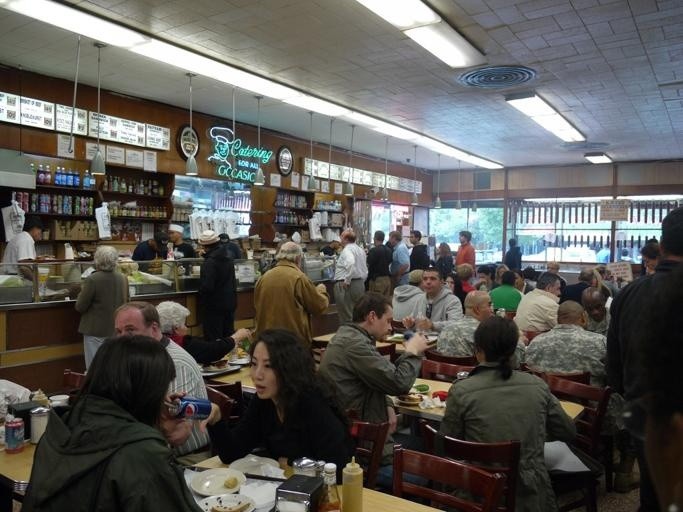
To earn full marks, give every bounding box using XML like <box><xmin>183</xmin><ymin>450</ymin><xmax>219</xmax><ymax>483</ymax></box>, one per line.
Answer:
<box><xmin>291</xmin><ymin>231</ymin><xmax>302</xmax><ymax>244</ymax></box>
<box><xmin>408</xmin><ymin>268</ymin><xmax>425</xmax><ymax>284</ymax></box>
<box><xmin>168</xmin><ymin>223</ymin><xmax>185</xmax><ymax>234</ymax></box>
<box><xmin>197</xmin><ymin>230</ymin><xmax>221</xmax><ymax>245</ymax></box>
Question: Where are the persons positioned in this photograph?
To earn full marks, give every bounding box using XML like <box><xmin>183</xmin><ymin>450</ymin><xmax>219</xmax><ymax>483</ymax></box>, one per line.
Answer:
<box><xmin>640</xmin><ymin>242</ymin><xmax>659</xmax><ymax>274</ymax></box>
<box><xmin>199</xmin><ymin>327</ymin><xmax>355</xmax><ymax>485</ymax></box>
<box><xmin>473</xmin><ymin>264</ymin><xmax>522</xmax><ymax>312</ymax></box>
<box><xmin>435</xmin><ymin>242</ymin><xmax>453</xmax><ymax>279</ymax></box>
<box><xmin>389</xmin><ymin>231</ymin><xmax>410</xmax><ymax>286</ymax></box>
<box><xmin>219</xmin><ymin>232</ymin><xmax>241</xmax><ymax>259</ymax></box>
<box><xmin>456</xmin><ymin>263</ymin><xmax>476</xmax><ymax>291</ymax></box>
<box><xmin>402</xmin><ymin>269</ymin><xmax>461</xmax><ymax>334</ymax></box>
<box><xmin>504</xmin><ymin>239</ymin><xmax>522</xmax><ymax>270</ymax></box>
<box><xmin>593</xmin><ymin>266</ymin><xmax>620</xmax><ymax>297</ymax></box>
<box><xmin>75</xmin><ymin>246</ymin><xmax>131</xmax><ymax>372</ymax></box>
<box><xmin>367</xmin><ymin>229</ymin><xmax>393</xmax><ymax>297</ymax></box>
<box><xmin>20</xmin><ymin>336</ymin><xmax>203</xmax><ymax>512</ymax></box>
<box><xmin>114</xmin><ymin>301</ymin><xmax>209</xmax><ymax>458</ymax></box>
<box><xmin>445</xmin><ymin>271</ymin><xmax>464</xmax><ymax>305</ymax></box>
<box><xmin>454</xmin><ymin>231</ymin><xmax>475</xmax><ymax>267</ymax></box>
<box><xmin>409</xmin><ymin>230</ymin><xmax>427</xmax><ymax>271</ymax></box>
<box><xmin>525</xmin><ymin>300</ymin><xmax>607</xmax><ymax>409</ymax></box>
<box><xmin>438</xmin><ymin>317</ymin><xmax>577</xmax><ymax>512</ymax></box>
<box><xmin>334</xmin><ymin>231</ymin><xmax>369</xmax><ymax>326</ymax></box>
<box><xmin>437</xmin><ymin>291</ymin><xmax>524</xmax><ymax>370</ymax></box>
<box><xmin>320</xmin><ymin>238</ymin><xmax>341</xmax><ymax>256</ymax></box>
<box><xmin>197</xmin><ymin>230</ymin><xmax>238</xmax><ymax>336</ymax></box>
<box><xmin>513</xmin><ymin>272</ymin><xmax>561</xmax><ymax>332</ymax></box>
<box><xmin>546</xmin><ymin>261</ymin><xmax>567</xmax><ymax>301</ymax></box>
<box><xmin>581</xmin><ymin>288</ymin><xmax>610</xmax><ymax>336</ymax></box>
<box><xmin>318</xmin><ymin>292</ymin><xmax>430</xmax><ymax>486</ymax></box>
<box><xmin>163</xmin><ymin>223</ymin><xmax>199</xmax><ymax>276</ymax></box>
<box><xmin>2</xmin><ymin>218</ymin><xmax>44</xmax><ymax>275</ymax></box>
<box><xmin>391</xmin><ymin>269</ymin><xmax>428</xmax><ymax>322</ymax></box>
<box><xmin>132</xmin><ymin>231</ymin><xmax>166</xmax><ymax>261</ymax></box>
<box><xmin>563</xmin><ymin>268</ymin><xmax>594</xmax><ymax>306</ymax></box>
<box><xmin>156</xmin><ymin>301</ymin><xmax>253</xmax><ymax>366</ymax></box>
<box><xmin>253</xmin><ymin>243</ymin><xmax>330</xmax><ymax>350</ymax></box>
<box><xmin>605</xmin><ymin>206</ymin><xmax>683</xmax><ymax>511</ymax></box>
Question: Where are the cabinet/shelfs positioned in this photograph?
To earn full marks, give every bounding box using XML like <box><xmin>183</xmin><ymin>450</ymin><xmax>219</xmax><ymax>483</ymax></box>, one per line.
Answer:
<box><xmin>257</xmin><ymin>187</ymin><xmax>314</xmax><ymax>244</ymax></box>
<box><xmin>3</xmin><ymin>262</ymin><xmax>336</xmax><ymax>352</ymax></box>
<box><xmin>313</xmin><ymin>193</ymin><xmax>348</xmax><ymax>245</ymax></box>
<box><xmin>12</xmin><ymin>151</ymin><xmax>175</xmax><ymax>258</ymax></box>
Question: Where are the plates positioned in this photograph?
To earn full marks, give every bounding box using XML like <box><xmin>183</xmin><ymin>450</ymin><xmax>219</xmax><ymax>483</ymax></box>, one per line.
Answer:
<box><xmin>384</xmin><ymin>332</ymin><xmax>439</xmax><ymax>346</ymax></box>
<box><xmin>190</xmin><ymin>467</ymin><xmax>247</xmax><ymax>496</ymax></box>
<box><xmin>393</xmin><ymin>395</ymin><xmax>429</xmax><ymax>405</ymax></box>
<box><xmin>182</xmin><ymin>465</ymin><xmax>288</xmax><ymax>512</ymax></box>
<box><xmin>199</xmin><ymin>362</ymin><xmax>241</xmax><ymax>377</ymax></box>
<box><xmin>203</xmin><ymin>367</ymin><xmax>229</xmax><ymax>372</ymax></box>
<box><xmin>197</xmin><ymin>494</ymin><xmax>255</xmax><ymax>512</ymax></box>
<box><xmin>409</xmin><ymin>384</ymin><xmax>431</xmax><ymax>393</ymax></box>
<box><xmin>228</xmin><ymin>349</ymin><xmax>252</xmax><ymax>366</ymax></box>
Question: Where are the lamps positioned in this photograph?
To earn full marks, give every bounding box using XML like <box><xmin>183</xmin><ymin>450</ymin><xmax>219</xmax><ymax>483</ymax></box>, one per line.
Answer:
<box><xmin>472</xmin><ymin>165</ymin><xmax>477</xmax><ymax>211</ymax></box>
<box><xmin>397</xmin><ymin>23</ymin><xmax>488</xmax><ymax>69</ymax></box>
<box><xmin>381</xmin><ymin>135</ymin><xmax>391</xmax><ymax>201</ymax></box>
<box><xmin>411</xmin><ymin>143</ymin><xmax>418</xmax><ymax>204</ymax></box>
<box><xmin>435</xmin><ymin>153</ymin><xmax>441</xmax><ymax>209</ymax></box>
<box><xmin>584</xmin><ymin>153</ymin><xmax>613</xmax><ymax>164</ymax></box>
<box><xmin>505</xmin><ymin>92</ymin><xmax>586</xmax><ymax>143</ymax></box>
<box><xmin>456</xmin><ymin>160</ymin><xmax>461</xmax><ymax>209</ymax></box>
<box><xmin>91</xmin><ymin>42</ymin><xmax>106</xmax><ymax>175</ymax></box>
<box><xmin>254</xmin><ymin>95</ymin><xmax>265</xmax><ymax>186</ymax></box>
<box><xmin>345</xmin><ymin>124</ymin><xmax>356</xmax><ymax>195</ymax></box>
<box><xmin>307</xmin><ymin>111</ymin><xmax>316</xmax><ymax>190</ymax></box>
<box><xmin>186</xmin><ymin>71</ymin><xmax>198</xmax><ymax>176</ymax></box>
<box><xmin>356</xmin><ymin>0</ymin><xmax>441</xmax><ymax>31</ymax></box>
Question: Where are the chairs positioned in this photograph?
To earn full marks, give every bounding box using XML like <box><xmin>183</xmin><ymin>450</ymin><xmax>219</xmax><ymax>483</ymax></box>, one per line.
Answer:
<box><xmin>390</xmin><ymin>320</ymin><xmax>415</xmax><ymax>334</ymax></box>
<box><xmin>522</xmin><ymin>330</ymin><xmax>550</xmax><ymax>344</ymax></box>
<box><xmin>63</xmin><ymin>369</ymin><xmax>86</xmax><ymax>393</ymax></box>
<box><xmin>207</xmin><ymin>381</ymin><xmax>245</xmax><ymax>419</ymax></box>
<box><xmin>504</xmin><ymin>311</ymin><xmax>516</xmax><ymax>319</ymax></box>
<box><xmin>541</xmin><ymin>371</ymin><xmax>613</xmax><ymax>493</ymax></box>
<box><xmin>421</xmin><ymin>359</ymin><xmax>476</xmax><ymax>384</ymax></box>
<box><xmin>202</xmin><ymin>385</ymin><xmax>242</xmax><ymax>464</ymax></box>
<box><xmin>424</xmin><ymin>350</ymin><xmax>476</xmax><ymax>367</ymax></box>
<box><xmin>348</xmin><ymin>420</ymin><xmax>391</xmax><ymax>486</ymax></box>
<box><xmin>420</xmin><ymin>425</ymin><xmax>522</xmax><ymax>512</ymax></box>
<box><xmin>377</xmin><ymin>343</ymin><xmax>396</xmax><ymax>366</ymax></box>
<box><xmin>519</xmin><ymin>361</ymin><xmax>591</xmax><ymax>408</ymax></box>
<box><xmin>392</xmin><ymin>442</ymin><xmax>503</xmax><ymax>512</ymax></box>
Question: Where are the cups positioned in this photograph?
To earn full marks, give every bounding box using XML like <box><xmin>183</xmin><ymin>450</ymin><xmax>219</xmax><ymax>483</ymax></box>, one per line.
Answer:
<box><xmin>50</xmin><ymin>394</ymin><xmax>68</xmax><ymax>409</ymax></box>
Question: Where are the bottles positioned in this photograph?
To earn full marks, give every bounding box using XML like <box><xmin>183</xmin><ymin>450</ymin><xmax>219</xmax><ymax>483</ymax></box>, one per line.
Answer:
<box><xmin>36</xmin><ymin>164</ymin><xmax>44</xmax><ymax>185</ymax></box>
<box><xmin>30</xmin><ymin>407</ymin><xmax>50</xmax><ymax>445</ymax></box>
<box><xmin>168</xmin><ymin>396</ymin><xmax>211</xmax><ymax>420</ymax></box>
<box><xmin>30</xmin><ymin>163</ymin><xmax>36</xmax><ymax>185</ymax></box>
<box><xmin>44</xmin><ymin>165</ymin><xmax>52</xmax><ymax>185</ymax></box>
<box><xmin>16</xmin><ymin>191</ymin><xmax>93</xmax><ymax>216</ymax></box>
<box><xmin>66</xmin><ymin>167</ymin><xmax>73</xmax><ymax>187</ymax></box>
<box><xmin>103</xmin><ymin>174</ymin><xmax>164</xmax><ymax>196</ymax></box>
<box><xmin>61</xmin><ymin>167</ymin><xmax>66</xmax><ymax>187</ymax></box>
<box><xmin>108</xmin><ymin>203</ymin><xmax>167</xmax><ymax>218</ymax></box>
<box><xmin>32</xmin><ymin>388</ymin><xmax>48</xmax><ymax>407</ymax></box>
<box><xmin>73</xmin><ymin>168</ymin><xmax>80</xmax><ymax>188</ymax></box>
<box><xmin>54</xmin><ymin>166</ymin><xmax>61</xmax><ymax>185</ymax></box>
<box><xmin>4</xmin><ymin>417</ymin><xmax>24</xmax><ymax>454</ymax></box>
<box><xmin>82</xmin><ymin>169</ymin><xmax>90</xmax><ymax>190</ymax></box>
<box><xmin>318</xmin><ymin>463</ymin><xmax>341</xmax><ymax>512</ymax></box>
<box><xmin>342</xmin><ymin>457</ymin><xmax>364</xmax><ymax>512</ymax></box>
<box><xmin>90</xmin><ymin>174</ymin><xmax>97</xmax><ymax>190</ymax></box>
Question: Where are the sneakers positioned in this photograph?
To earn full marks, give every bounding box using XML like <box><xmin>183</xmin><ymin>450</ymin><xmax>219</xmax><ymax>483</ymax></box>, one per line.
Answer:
<box><xmin>613</xmin><ymin>471</ymin><xmax>641</xmax><ymax>493</ymax></box>
